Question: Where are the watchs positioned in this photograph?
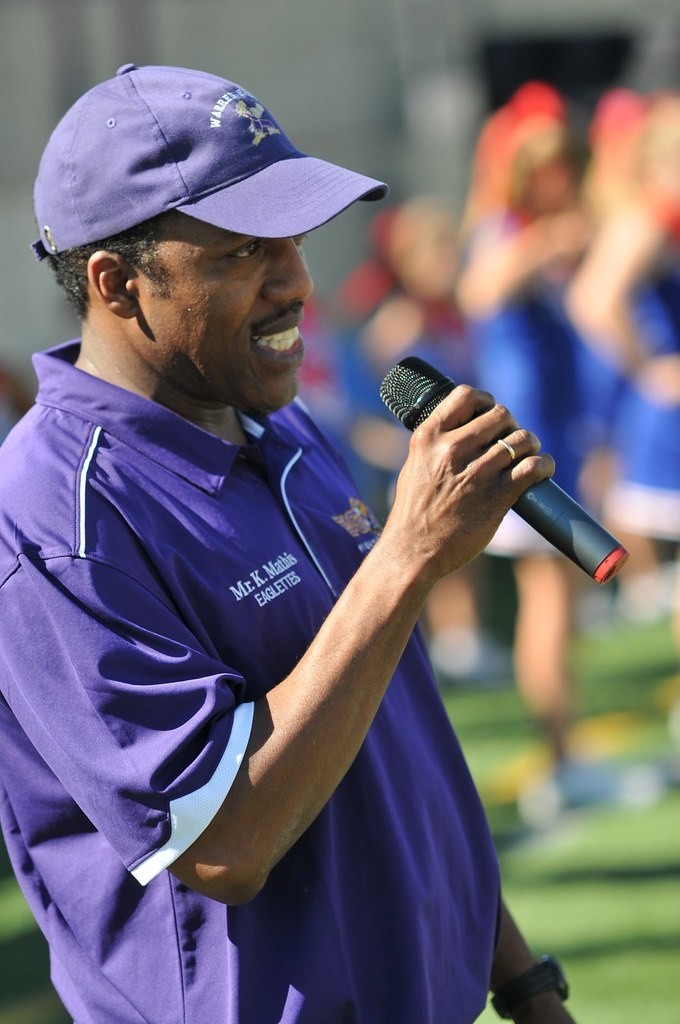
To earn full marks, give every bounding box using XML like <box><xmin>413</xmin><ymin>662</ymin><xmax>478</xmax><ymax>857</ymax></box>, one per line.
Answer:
<box><xmin>491</xmin><ymin>952</ymin><xmax>570</xmax><ymax>1019</ymax></box>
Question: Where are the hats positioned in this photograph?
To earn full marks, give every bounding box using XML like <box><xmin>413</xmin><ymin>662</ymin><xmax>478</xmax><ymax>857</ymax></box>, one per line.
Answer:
<box><xmin>31</xmin><ymin>62</ymin><xmax>388</xmax><ymax>263</ymax></box>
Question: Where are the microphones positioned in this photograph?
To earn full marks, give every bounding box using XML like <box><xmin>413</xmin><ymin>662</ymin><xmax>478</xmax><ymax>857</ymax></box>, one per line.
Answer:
<box><xmin>378</xmin><ymin>355</ymin><xmax>630</xmax><ymax>585</ymax></box>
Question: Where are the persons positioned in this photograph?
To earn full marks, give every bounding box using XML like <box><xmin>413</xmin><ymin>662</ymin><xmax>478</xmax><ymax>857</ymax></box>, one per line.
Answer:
<box><xmin>0</xmin><ymin>61</ymin><xmax>577</xmax><ymax>1024</ymax></box>
<box><xmin>299</xmin><ymin>79</ymin><xmax>680</xmax><ymax>829</ymax></box>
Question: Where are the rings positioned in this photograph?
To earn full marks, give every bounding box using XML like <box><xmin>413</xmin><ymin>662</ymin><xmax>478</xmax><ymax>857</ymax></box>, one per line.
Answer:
<box><xmin>497</xmin><ymin>439</ymin><xmax>515</xmax><ymax>462</ymax></box>
